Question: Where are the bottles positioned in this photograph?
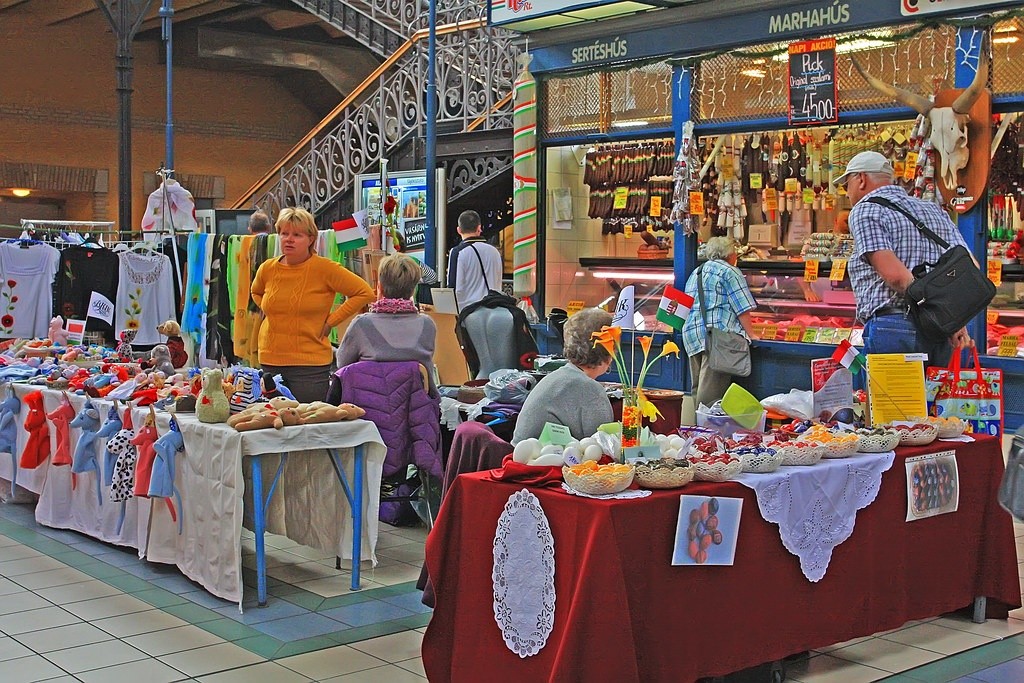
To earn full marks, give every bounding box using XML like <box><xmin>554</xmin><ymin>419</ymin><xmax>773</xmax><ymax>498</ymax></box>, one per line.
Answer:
<box><xmin>368</xmin><ymin>192</ymin><xmax>426</xmax><ymax>225</ymax></box>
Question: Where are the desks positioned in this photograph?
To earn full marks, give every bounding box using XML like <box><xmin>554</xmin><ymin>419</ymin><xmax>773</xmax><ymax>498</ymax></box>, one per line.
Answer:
<box><xmin>1</xmin><ymin>382</ymin><xmax>387</xmax><ymax>617</ymax></box>
<box><xmin>598</xmin><ymin>380</ymin><xmax>684</xmax><ymax>436</ymax></box>
<box><xmin>421</xmin><ymin>433</ymin><xmax>1022</xmax><ymax>683</ymax></box>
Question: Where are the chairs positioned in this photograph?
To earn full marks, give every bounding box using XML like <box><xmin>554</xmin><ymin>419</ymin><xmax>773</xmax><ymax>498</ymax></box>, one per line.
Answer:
<box><xmin>417</xmin><ymin>420</ymin><xmax>515</xmax><ymax>609</ymax></box>
<box><xmin>325</xmin><ymin>361</ymin><xmax>443</xmax><ymax>569</ymax></box>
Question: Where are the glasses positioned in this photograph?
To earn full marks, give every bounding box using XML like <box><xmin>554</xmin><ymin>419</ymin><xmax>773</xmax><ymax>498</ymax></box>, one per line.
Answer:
<box><xmin>841</xmin><ymin>173</ymin><xmax>859</xmax><ymax>192</ymax></box>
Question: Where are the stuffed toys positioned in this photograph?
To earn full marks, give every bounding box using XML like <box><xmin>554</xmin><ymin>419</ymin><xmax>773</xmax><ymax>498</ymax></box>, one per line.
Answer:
<box><xmin>0</xmin><ymin>316</ymin><xmax>365</xmax><ymax>537</ymax></box>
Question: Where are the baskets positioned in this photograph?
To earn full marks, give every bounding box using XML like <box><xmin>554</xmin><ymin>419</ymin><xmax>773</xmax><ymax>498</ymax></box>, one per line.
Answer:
<box><xmin>858</xmin><ymin>415</ymin><xmax>967</xmax><ymax>453</ymax></box>
<box><xmin>562</xmin><ymin>452</ymin><xmax>742</xmax><ymax>495</ymax></box>
<box><xmin>741</xmin><ymin>425</ymin><xmax>860</xmax><ymax>473</ymax></box>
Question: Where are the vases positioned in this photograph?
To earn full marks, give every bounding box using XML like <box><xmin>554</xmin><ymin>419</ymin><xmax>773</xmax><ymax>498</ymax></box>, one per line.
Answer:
<box><xmin>622</xmin><ymin>398</ymin><xmax>640</xmax><ymax>448</ymax></box>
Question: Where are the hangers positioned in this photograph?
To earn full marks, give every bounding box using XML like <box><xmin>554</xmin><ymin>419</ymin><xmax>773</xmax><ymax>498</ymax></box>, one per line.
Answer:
<box><xmin>0</xmin><ymin>226</ymin><xmax>163</xmax><ymax>257</ymax></box>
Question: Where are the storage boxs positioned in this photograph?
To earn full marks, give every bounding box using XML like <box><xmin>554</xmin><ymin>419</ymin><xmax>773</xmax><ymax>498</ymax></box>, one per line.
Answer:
<box><xmin>694</xmin><ymin>408</ymin><xmax>767</xmax><ymax>436</ymax></box>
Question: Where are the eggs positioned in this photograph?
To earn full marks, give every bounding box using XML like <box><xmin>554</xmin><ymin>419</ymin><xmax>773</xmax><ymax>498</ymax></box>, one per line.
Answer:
<box><xmin>513</xmin><ymin>437</ymin><xmax>601</xmax><ymax>466</ymax></box>
<box><xmin>656</xmin><ymin>434</ymin><xmax>685</xmax><ymax>458</ymax></box>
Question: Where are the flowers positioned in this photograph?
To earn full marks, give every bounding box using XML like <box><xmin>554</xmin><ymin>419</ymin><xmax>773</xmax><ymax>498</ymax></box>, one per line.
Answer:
<box><xmin>589</xmin><ymin>326</ymin><xmax>680</xmax><ymax>447</ymax></box>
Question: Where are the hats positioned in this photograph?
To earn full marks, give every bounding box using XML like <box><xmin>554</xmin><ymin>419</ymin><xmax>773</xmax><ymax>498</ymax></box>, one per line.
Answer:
<box><xmin>542</xmin><ymin>308</ymin><xmax>568</xmax><ymax>319</ymax></box>
<box><xmin>833</xmin><ymin>151</ymin><xmax>893</xmax><ymax>183</ymax></box>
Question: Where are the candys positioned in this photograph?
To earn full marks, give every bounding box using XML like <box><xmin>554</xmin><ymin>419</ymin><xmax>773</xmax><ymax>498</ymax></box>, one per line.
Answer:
<box><xmin>912</xmin><ymin>463</ymin><xmax>952</xmax><ymax>512</ymax></box>
<box><xmin>567</xmin><ymin>460</ymin><xmax>691</xmax><ymax>476</ymax></box>
<box><xmin>688</xmin><ymin>498</ymin><xmax>722</xmax><ymax>563</ymax></box>
<box><xmin>687</xmin><ymin>418</ymin><xmax>934</xmax><ymax>465</ymax></box>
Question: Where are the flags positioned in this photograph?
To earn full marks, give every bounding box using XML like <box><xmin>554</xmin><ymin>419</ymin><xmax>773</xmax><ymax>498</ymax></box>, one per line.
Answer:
<box><xmin>656</xmin><ymin>286</ymin><xmax>694</xmax><ymax>332</ymax></box>
<box><xmin>332</xmin><ymin>208</ymin><xmax>370</xmax><ymax>252</ymax></box>
<box><xmin>612</xmin><ymin>286</ymin><xmax>635</xmax><ymax>330</ymax></box>
<box><xmin>87</xmin><ymin>292</ymin><xmax>114</xmax><ymax>326</ymax></box>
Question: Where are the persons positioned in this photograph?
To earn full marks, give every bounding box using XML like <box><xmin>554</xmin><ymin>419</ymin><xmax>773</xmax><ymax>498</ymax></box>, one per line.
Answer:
<box><xmin>336</xmin><ymin>252</ymin><xmax>437</xmax><ymax>382</ymax></box>
<box><xmin>681</xmin><ymin>236</ymin><xmax>759</xmax><ymax>411</ymax></box>
<box><xmin>510</xmin><ymin>310</ymin><xmax>614</xmax><ymax>449</ymax></box>
<box><xmin>247</xmin><ymin>212</ymin><xmax>270</xmax><ymax>235</ymax></box>
<box><xmin>446</xmin><ymin>210</ymin><xmax>504</xmax><ymax>313</ymax></box>
<box><xmin>833</xmin><ymin>151</ymin><xmax>976</xmax><ymax>394</ymax></box>
<box><xmin>251</xmin><ymin>208</ymin><xmax>375</xmax><ymax>404</ymax></box>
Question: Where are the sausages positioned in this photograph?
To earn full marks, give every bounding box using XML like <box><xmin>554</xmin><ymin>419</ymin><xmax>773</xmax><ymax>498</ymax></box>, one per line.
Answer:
<box><xmin>583</xmin><ymin>140</ymin><xmax>675</xmax><ymax>235</ymax></box>
<box><xmin>1016</xmin><ymin>113</ymin><xmax>1024</xmax><ymax>221</ymax></box>
<box><xmin>702</xmin><ymin>113</ymin><xmax>935</xmax><ymax>255</ymax></box>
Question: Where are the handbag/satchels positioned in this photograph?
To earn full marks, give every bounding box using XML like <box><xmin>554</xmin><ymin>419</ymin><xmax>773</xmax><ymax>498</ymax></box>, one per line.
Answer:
<box><xmin>705</xmin><ymin>327</ymin><xmax>752</xmax><ymax>377</ymax></box>
<box><xmin>998</xmin><ymin>424</ymin><xmax>1024</xmax><ymax>523</ymax></box>
<box><xmin>904</xmin><ymin>244</ymin><xmax>997</xmax><ymax>343</ymax></box>
<box><xmin>925</xmin><ymin>345</ymin><xmax>1004</xmax><ymax>449</ymax></box>
<box><xmin>483</xmin><ymin>369</ymin><xmax>537</xmax><ymax>404</ymax></box>
<box><xmin>380</xmin><ymin>472</ymin><xmax>421</xmax><ymax>526</ymax></box>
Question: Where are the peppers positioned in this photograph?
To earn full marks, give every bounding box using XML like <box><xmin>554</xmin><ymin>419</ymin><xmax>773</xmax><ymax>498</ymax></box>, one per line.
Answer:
<box><xmin>991</xmin><ymin>119</ymin><xmax>1020</xmax><ymax>199</ymax></box>
